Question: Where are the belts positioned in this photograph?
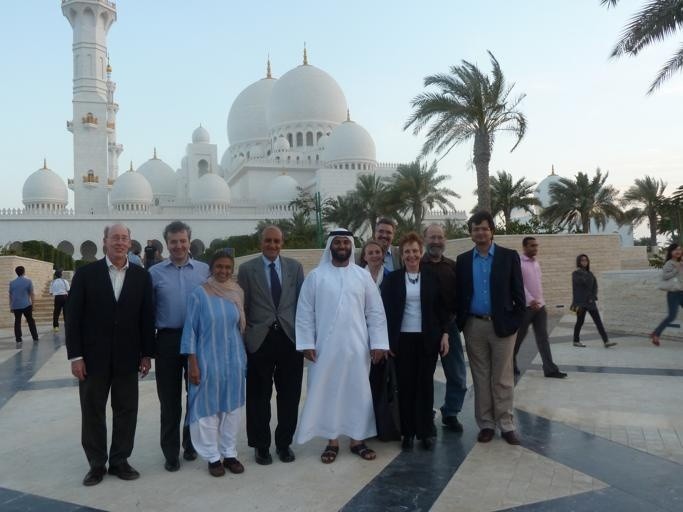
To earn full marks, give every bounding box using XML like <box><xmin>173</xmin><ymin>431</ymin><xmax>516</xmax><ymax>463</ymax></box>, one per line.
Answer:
<box><xmin>475</xmin><ymin>314</ymin><xmax>492</xmax><ymax>320</ymax></box>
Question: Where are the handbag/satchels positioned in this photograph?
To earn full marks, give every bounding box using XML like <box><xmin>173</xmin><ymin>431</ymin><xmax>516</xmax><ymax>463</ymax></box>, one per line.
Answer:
<box><xmin>569</xmin><ymin>304</ymin><xmax>582</xmax><ymax>312</ymax></box>
<box><xmin>656</xmin><ymin>277</ymin><xmax>680</xmax><ymax>292</ymax></box>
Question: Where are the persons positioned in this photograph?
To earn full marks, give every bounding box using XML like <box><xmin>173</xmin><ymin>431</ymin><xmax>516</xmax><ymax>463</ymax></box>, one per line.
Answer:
<box><xmin>128</xmin><ymin>237</ymin><xmax>212</xmax><ymax>269</ymax></box>
<box><xmin>292</xmin><ymin>227</ymin><xmax>390</xmax><ymax>464</ymax></box>
<box><xmin>64</xmin><ymin>223</ymin><xmax>154</xmax><ymax>487</ymax></box>
<box><xmin>452</xmin><ymin>210</ymin><xmax>526</xmax><ymax>445</ymax></box>
<box><xmin>8</xmin><ymin>266</ymin><xmax>38</xmax><ymax>342</ymax></box>
<box><xmin>649</xmin><ymin>243</ymin><xmax>683</xmax><ymax>346</ymax></box>
<box><xmin>571</xmin><ymin>253</ymin><xmax>616</xmax><ymax>348</ymax></box>
<box><xmin>147</xmin><ymin>221</ymin><xmax>208</xmax><ymax>470</ymax></box>
<box><xmin>362</xmin><ymin>219</ymin><xmax>467</xmax><ymax>451</ymax></box>
<box><xmin>234</xmin><ymin>225</ymin><xmax>305</xmax><ymax>466</ymax></box>
<box><xmin>49</xmin><ymin>269</ymin><xmax>69</xmax><ymax>331</ymax></box>
<box><xmin>513</xmin><ymin>237</ymin><xmax>567</xmax><ymax>379</ymax></box>
<box><xmin>179</xmin><ymin>247</ymin><xmax>247</xmax><ymax>477</ymax></box>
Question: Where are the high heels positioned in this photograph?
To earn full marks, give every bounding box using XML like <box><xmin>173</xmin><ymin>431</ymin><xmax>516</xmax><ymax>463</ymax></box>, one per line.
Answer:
<box><xmin>648</xmin><ymin>331</ymin><xmax>660</xmax><ymax>347</ymax></box>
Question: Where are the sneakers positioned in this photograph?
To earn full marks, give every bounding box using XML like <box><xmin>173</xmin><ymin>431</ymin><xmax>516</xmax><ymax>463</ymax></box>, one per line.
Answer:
<box><xmin>573</xmin><ymin>342</ymin><xmax>585</xmax><ymax>347</ymax></box>
<box><xmin>604</xmin><ymin>342</ymin><xmax>616</xmax><ymax>348</ymax></box>
<box><xmin>16</xmin><ymin>326</ymin><xmax>60</xmax><ymax>343</ymax></box>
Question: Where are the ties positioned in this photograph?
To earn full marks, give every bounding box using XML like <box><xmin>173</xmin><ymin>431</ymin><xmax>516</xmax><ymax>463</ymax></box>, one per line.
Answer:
<box><xmin>268</xmin><ymin>262</ymin><xmax>281</xmax><ymax>304</ymax></box>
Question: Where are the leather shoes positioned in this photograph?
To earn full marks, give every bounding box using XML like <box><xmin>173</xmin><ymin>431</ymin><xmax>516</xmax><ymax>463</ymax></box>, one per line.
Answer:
<box><xmin>165</xmin><ymin>444</ymin><xmax>296</xmax><ymax>476</ymax></box>
<box><xmin>501</xmin><ymin>432</ymin><xmax>521</xmax><ymax>445</ymax></box>
<box><xmin>377</xmin><ymin>413</ymin><xmax>463</xmax><ymax>453</ymax></box>
<box><xmin>544</xmin><ymin>371</ymin><xmax>567</xmax><ymax>378</ymax></box>
<box><xmin>477</xmin><ymin>428</ymin><xmax>494</xmax><ymax>442</ymax></box>
<box><xmin>108</xmin><ymin>463</ymin><xmax>140</xmax><ymax>480</ymax></box>
<box><xmin>513</xmin><ymin>366</ymin><xmax>520</xmax><ymax>375</ymax></box>
<box><xmin>84</xmin><ymin>465</ymin><xmax>108</xmax><ymax>486</ymax></box>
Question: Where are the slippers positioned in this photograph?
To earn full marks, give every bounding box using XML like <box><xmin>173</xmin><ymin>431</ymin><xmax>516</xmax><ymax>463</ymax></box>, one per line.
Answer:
<box><xmin>350</xmin><ymin>444</ymin><xmax>376</xmax><ymax>461</ymax></box>
<box><xmin>320</xmin><ymin>443</ymin><xmax>339</xmax><ymax>463</ymax></box>
